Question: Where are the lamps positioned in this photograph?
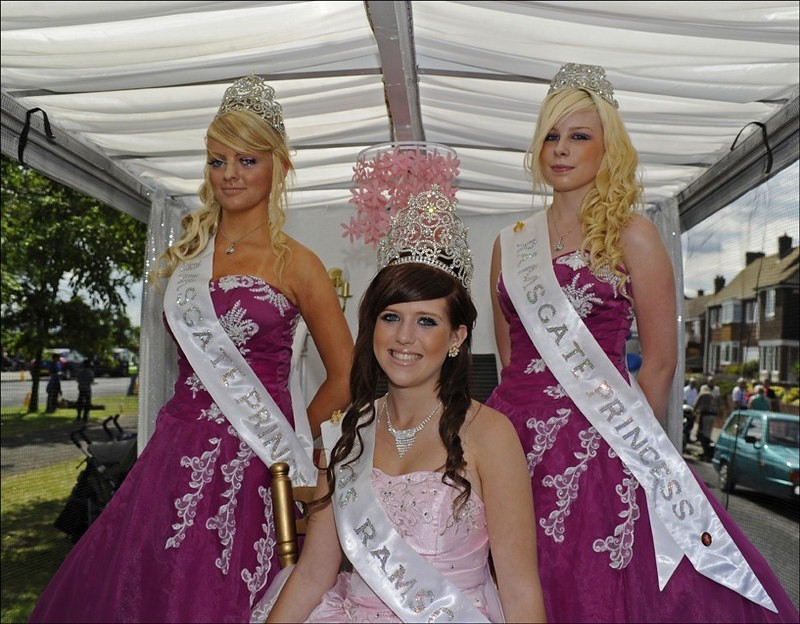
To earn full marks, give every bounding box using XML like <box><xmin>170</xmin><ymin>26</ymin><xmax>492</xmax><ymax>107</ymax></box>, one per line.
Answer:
<box><xmin>328</xmin><ymin>265</ymin><xmax>353</xmax><ymax>313</ymax></box>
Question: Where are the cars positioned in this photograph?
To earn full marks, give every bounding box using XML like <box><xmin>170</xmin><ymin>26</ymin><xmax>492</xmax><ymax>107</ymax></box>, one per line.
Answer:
<box><xmin>711</xmin><ymin>408</ymin><xmax>800</xmax><ymax>505</ymax></box>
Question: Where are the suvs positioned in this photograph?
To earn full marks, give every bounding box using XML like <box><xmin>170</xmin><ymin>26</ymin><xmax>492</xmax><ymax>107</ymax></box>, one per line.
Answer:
<box><xmin>30</xmin><ymin>348</ymin><xmax>85</xmax><ymax>380</ymax></box>
<box><xmin>94</xmin><ymin>348</ymin><xmax>138</xmax><ymax>376</ymax></box>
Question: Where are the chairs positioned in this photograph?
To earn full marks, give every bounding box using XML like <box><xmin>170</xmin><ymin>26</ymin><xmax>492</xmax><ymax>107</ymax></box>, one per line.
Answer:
<box><xmin>269</xmin><ymin>461</ymin><xmax>316</xmax><ymax>569</ymax></box>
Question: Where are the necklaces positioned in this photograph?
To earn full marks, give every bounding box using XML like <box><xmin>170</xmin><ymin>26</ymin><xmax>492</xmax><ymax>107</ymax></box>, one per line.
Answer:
<box><xmin>219</xmin><ymin>225</ymin><xmax>263</xmax><ymax>255</ymax></box>
<box><xmin>551</xmin><ymin>204</ymin><xmax>578</xmax><ymax>251</ymax></box>
<box><xmin>385</xmin><ymin>400</ymin><xmax>442</xmax><ymax>458</ymax></box>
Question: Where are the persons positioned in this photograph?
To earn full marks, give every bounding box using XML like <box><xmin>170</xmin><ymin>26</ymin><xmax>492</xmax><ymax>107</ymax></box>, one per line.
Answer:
<box><xmin>684</xmin><ymin>377</ymin><xmax>779</xmax><ymax>459</ymax></box>
<box><xmin>481</xmin><ymin>62</ymin><xmax>800</xmax><ymax>624</ymax></box>
<box><xmin>44</xmin><ymin>353</ymin><xmax>94</xmax><ymax>420</ymax></box>
<box><xmin>264</xmin><ymin>184</ymin><xmax>547</xmax><ymax>624</ymax></box>
<box><xmin>26</xmin><ymin>73</ymin><xmax>356</xmax><ymax>624</ymax></box>
